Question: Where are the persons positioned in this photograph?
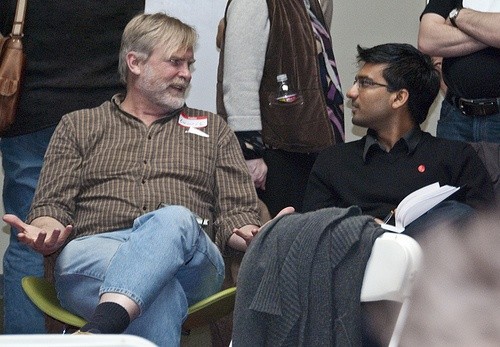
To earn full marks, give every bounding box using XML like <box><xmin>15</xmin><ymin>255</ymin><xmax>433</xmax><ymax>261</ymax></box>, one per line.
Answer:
<box><xmin>216</xmin><ymin>0</ymin><xmax>345</xmax><ymax>221</ymax></box>
<box><xmin>303</xmin><ymin>43</ymin><xmax>495</xmax><ymax>226</ymax></box>
<box><xmin>3</xmin><ymin>13</ymin><xmax>296</xmax><ymax>347</ymax></box>
<box><xmin>0</xmin><ymin>0</ymin><xmax>145</xmax><ymax>334</ymax></box>
<box><xmin>418</xmin><ymin>0</ymin><xmax>500</xmax><ymax>142</ymax></box>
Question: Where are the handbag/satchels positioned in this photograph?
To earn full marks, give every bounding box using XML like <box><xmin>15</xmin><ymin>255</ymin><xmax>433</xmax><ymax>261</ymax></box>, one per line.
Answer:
<box><xmin>0</xmin><ymin>0</ymin><xmax>27</xmax><ymax>130</ymax></box>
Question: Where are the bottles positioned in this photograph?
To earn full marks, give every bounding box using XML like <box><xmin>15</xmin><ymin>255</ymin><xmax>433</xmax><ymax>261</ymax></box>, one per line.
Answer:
<box><xmin>275</xmin><ymin>74</ymin><xmax>297</xmax><ymax>104</ymax></box>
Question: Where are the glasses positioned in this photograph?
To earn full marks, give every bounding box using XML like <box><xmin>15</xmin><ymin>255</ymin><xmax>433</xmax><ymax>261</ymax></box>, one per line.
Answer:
<box><xmin>353</xmin><ymin>78</ymin><xmax>394</xmax><ymax>92</ymax></box>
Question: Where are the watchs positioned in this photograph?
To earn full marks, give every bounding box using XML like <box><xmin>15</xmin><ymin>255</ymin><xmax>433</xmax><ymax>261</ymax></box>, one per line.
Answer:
<box><xmin>449</xmin><ymin>7</ymin><xmax>465</xmax><ymax>27</ymax></box>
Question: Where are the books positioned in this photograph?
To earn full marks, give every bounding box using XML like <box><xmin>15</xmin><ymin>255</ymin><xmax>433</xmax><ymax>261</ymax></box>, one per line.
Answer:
<box><xmin>374</xmin><ymin>182</ymin><xmax>461</xmax><ymax>233</ymax></box>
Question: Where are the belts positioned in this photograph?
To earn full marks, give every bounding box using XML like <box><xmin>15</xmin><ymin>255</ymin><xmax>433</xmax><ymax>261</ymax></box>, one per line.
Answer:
<box><xmin>445</xmin><ymin>89</ymin><xmax>500</xmax><ymax>117</ymax></box>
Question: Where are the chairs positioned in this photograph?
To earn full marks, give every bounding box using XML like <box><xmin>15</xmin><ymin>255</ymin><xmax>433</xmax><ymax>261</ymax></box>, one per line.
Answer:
<box><xmin>228</xmin><ymin>232</ymin><xmax>422</xmax><ymax>347</ymax></box>
<box><xmin>21</xmin><ymin>276</ymin><xmax>236</xmax><ymax>334</ymax></box>
